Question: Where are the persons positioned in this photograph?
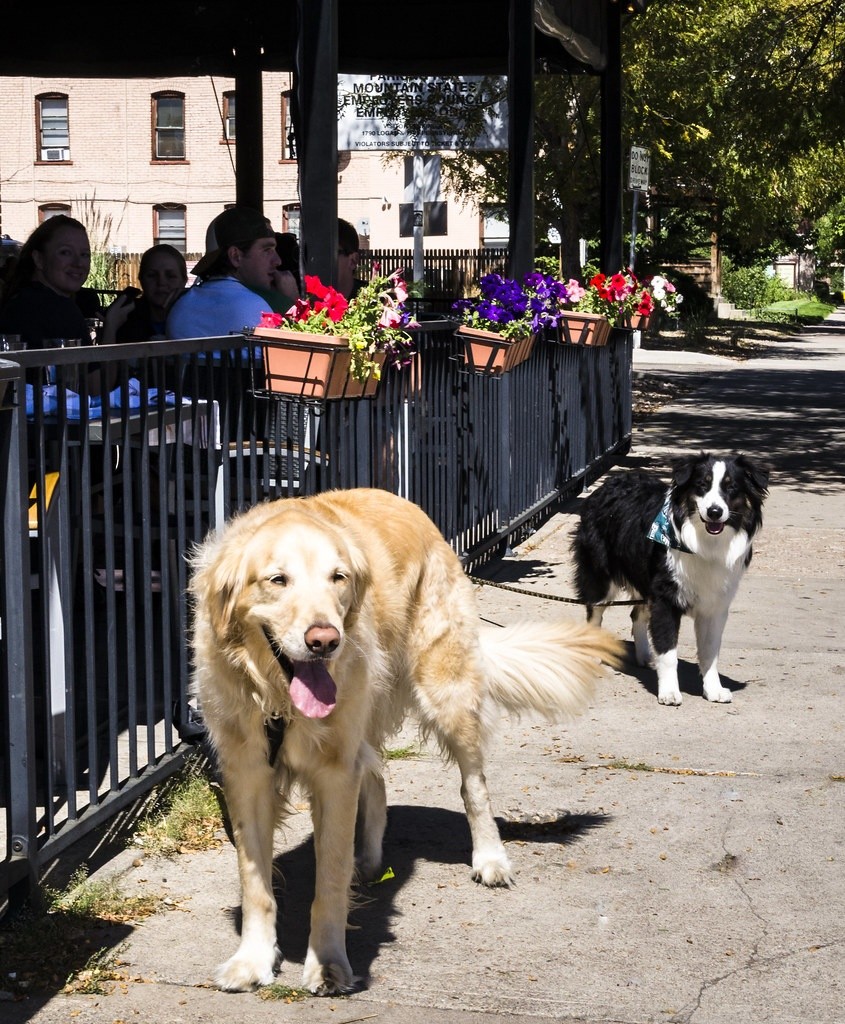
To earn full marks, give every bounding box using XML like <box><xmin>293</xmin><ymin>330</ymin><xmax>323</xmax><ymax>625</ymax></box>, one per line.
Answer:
<box><xmin>0</xmin><ymin>214</ymin><xmax>363</xmax><ymax>387</ymax></box>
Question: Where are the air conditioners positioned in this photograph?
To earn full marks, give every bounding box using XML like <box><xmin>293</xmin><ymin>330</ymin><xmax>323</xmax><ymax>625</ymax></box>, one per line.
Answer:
<box><xmin>41</xmin><ymin>148</ymin><xmax>65</xmax><ymax>162</ymax></box>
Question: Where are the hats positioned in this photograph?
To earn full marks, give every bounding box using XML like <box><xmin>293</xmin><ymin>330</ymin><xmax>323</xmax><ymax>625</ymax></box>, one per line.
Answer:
<box><xmin>190</xmin><ymin>208</ymin><xmax>271</xmax><ymax>275</ymax></box>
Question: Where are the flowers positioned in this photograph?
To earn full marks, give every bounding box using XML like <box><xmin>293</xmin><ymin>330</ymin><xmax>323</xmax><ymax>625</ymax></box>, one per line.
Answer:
<box><xmin>452</xmin><ymin>272</ymin><xmax>570</xmax><ymax>348</ymax></box>
<box><xmin>251</xmin><ymin>271</ymin><xmax>422</xmax><ymax>385</ymax></box>
<box><xmin>557</xmin><ymin>269</ymin><xmax>655</xmax><ymax>326</ymax></box>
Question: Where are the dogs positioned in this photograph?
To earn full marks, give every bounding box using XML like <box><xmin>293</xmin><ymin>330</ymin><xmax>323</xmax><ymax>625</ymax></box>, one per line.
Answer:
<box><xmin>571</xmin><ymin>451</ymin><xmax>779</xmax><ymax>706</ymax></box>
<box><xmin>182</xmin><ymin>488</ymin><xmax>626</xmax><ymax>994</ymax></box>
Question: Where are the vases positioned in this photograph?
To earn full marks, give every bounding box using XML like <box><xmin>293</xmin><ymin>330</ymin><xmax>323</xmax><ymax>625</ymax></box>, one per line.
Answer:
<box><xmin>254</xmin><ymin>327</ymin><xmax>387</xmax><ymax>400</ymax></box>
<box><xmin>458</xmin><ymin>324</ymin><xmax>538</xmax><ymax>373</ymax></box>
<box><xmin>620</xmin><ymin>311</ymin><xmax>650</xmax><ymax>329</ymax></box>
<box><xmin>556</xmin><ymin>307</ymin><xmax>609</xmax><ymax>347</ymax></box>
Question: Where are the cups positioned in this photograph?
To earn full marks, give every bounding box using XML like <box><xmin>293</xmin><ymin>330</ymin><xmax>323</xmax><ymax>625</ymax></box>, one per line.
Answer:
<box><xmin>44</xmin><ymin>339</ymin><xmax>81</xmax><ymax>394</ymax></box>
<box><xmin>0</xmin><ymin>334</ymin><xmax>27</xmax><ymax>390</ymax></box>
